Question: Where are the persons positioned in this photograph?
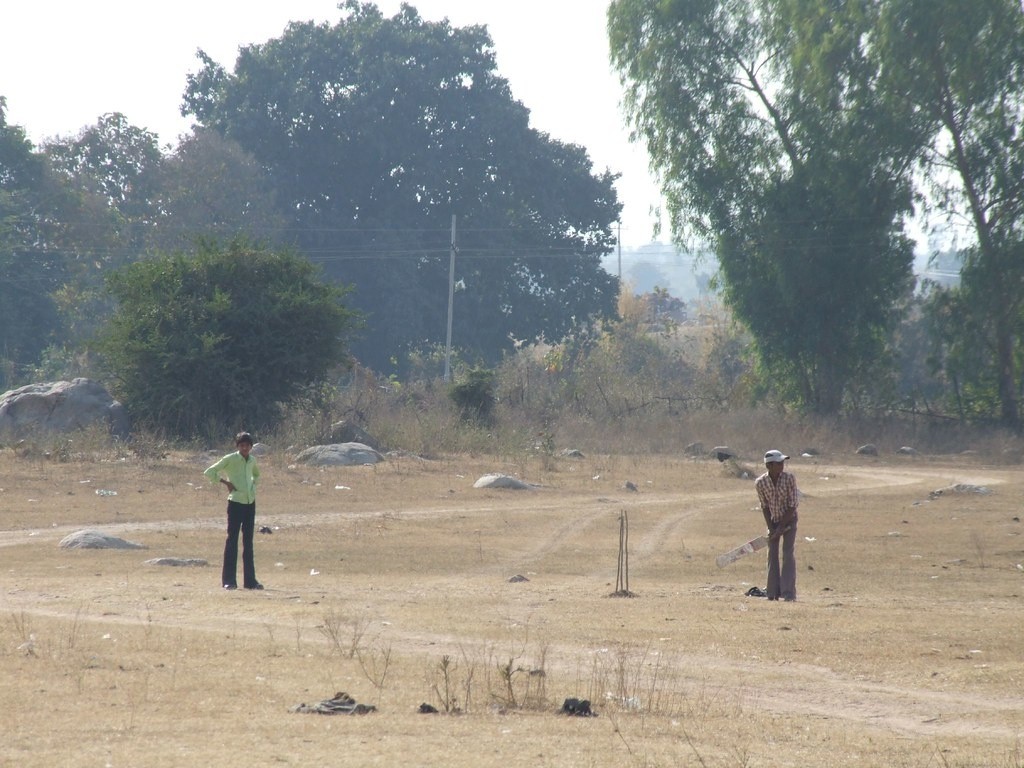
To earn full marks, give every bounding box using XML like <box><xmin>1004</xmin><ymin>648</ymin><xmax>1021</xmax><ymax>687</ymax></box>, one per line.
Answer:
<box><xmin>204</xmin><ymin>432</ymin><xmax>263</xmax><ymax>590</ymax></box>
<box><xmin>754</xmin><ymin>450</ymin><xmax>799</xmax><ymax>602</ymax></box>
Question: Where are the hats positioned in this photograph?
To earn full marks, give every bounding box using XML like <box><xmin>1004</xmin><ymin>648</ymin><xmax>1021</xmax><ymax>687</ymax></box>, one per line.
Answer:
<box><xmin>763</xmin><ymin>450</ymin><xmax>790</xmax><ymax>463</ymax></box>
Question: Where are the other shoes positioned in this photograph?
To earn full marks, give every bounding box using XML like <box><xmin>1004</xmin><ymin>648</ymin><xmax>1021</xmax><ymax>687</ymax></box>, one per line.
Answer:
<box><xmin>244</xmin><ymin>583</ymin><xmax>263</xmax><ymax>590</ymax></box>
<box><xmin>783</xmin><ymin>591</ymin><xmax>796</xmax><ymax>601</ymax></box>
<box><xmin>767</xmin><ymin>592</ymin><xmax>779</xmax><ymax>601</ymax></box>
<box><xmin>224</xmin><ymin>583</ymin><xmax>236</xmax><ymax>590</ymax></box>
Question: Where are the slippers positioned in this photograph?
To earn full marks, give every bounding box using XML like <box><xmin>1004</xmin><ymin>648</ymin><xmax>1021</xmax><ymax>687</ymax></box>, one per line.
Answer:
<box><xmin>745</xmin><ymin>587</ymin><xmax>764</xmax><ymax>596</ymax></box>
